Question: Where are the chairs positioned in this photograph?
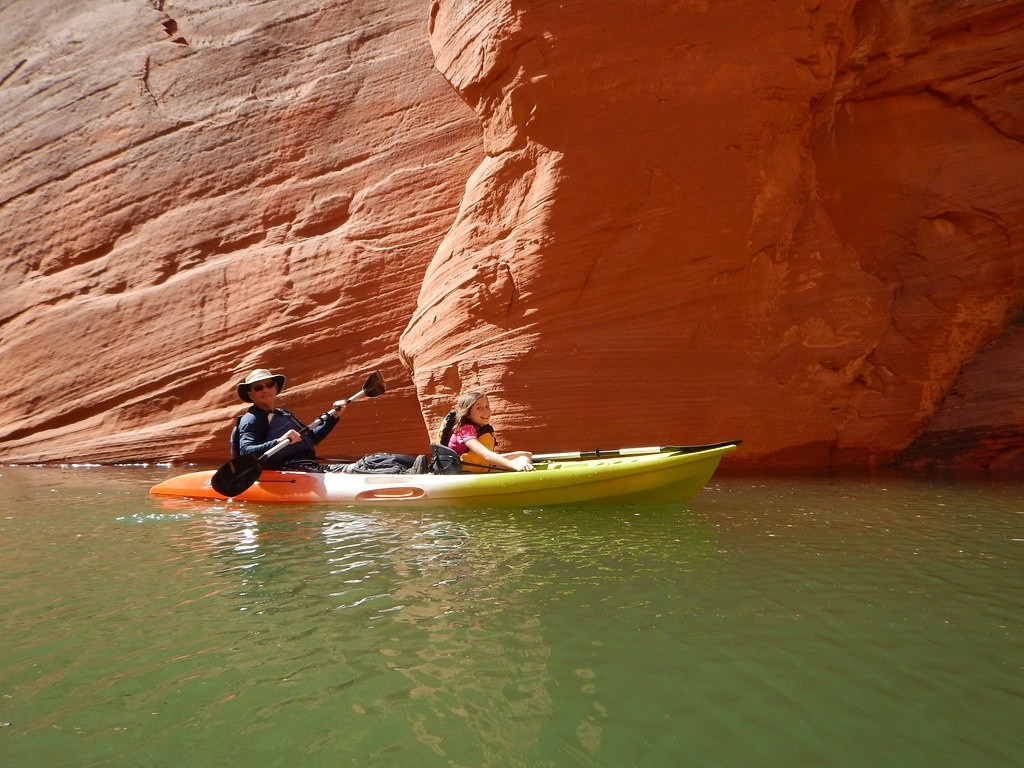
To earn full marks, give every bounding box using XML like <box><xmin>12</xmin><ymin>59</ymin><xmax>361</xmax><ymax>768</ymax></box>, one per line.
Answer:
<box><xmin>429</xmin><ymin>443</ymin><xmax>463</xmax><ymax>474</ymax></box>
<box><xmin>230</xmin><ymin>416</ymin><xmax>240</xmax><ymax>459</ymax></box>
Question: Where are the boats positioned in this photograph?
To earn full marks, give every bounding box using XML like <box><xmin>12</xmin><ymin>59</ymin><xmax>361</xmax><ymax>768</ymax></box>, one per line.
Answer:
<box><xmin>148</xmin><ymin>441</ymin><xmax>746</xmax><ymax>506</ymax></box>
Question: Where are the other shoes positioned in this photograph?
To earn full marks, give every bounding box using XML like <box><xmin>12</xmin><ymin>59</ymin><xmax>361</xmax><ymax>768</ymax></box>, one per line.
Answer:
<box><xmin>406</xmin><ymin>455</ymin><xmax>428</xmax><ymax>475</ymax></box>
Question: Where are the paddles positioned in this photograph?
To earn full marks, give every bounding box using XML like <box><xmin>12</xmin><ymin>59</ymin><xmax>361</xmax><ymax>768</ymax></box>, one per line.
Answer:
<box><xmin>531</xmin><ymin>439</ymin><xmax>745</xmax><ymax>463</ymax></box>
<box><xmin>210</xmin><ymin>370</ymin><xmax>387</xmax><ymax>498</ymax></box>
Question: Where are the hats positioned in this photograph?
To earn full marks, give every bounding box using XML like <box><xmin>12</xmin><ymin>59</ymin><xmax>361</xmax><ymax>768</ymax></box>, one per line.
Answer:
<box><xmin>236</xmin><ymin>368</ymin><xmax>287</xmax><ymax>403</ymax></box>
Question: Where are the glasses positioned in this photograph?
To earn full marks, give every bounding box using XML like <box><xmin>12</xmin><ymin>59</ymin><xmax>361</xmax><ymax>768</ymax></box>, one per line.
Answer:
<box><xmin>253</xmin><ymin>382</ymin><xmax>276</xmax><ymax>392</ymax></box>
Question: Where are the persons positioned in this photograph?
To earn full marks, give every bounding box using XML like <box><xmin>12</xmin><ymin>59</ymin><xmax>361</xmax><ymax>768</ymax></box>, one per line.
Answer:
<box><xmin>447</xmin><ymin>390</ymin><xmax>537</xmax><ymax>473</ymax></box>
<box><xmin>235</xmin><ymin>369</ymin><xmax>428</xmax><ymax>474</ymax></box>
